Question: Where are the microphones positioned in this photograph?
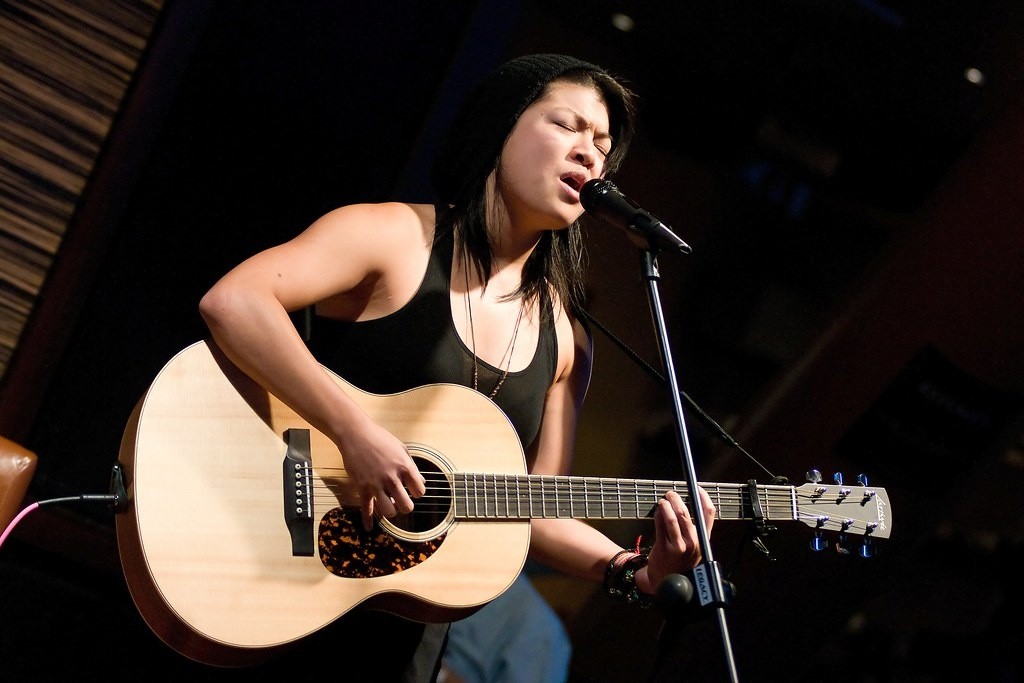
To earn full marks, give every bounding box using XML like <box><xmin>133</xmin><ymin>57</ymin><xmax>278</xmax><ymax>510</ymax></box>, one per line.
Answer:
<box><xmin>578</xmin><ymin>176</ymin><xmax>695</xmax><ymax>258</ymax></box>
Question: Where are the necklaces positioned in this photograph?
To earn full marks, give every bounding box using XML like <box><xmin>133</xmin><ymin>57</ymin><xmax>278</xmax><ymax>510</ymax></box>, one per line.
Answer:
<box><xmin>452</xmin><ymin>204</ymin><xmax>527</xmax><ymax>404</ymax></box>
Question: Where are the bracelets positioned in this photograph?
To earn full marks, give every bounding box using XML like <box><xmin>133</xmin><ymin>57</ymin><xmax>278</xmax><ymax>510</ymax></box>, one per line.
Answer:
<box><xmin>603</xmin><ymin>549</ymin><xmax>655</xmax><ymax>611</ymax></box>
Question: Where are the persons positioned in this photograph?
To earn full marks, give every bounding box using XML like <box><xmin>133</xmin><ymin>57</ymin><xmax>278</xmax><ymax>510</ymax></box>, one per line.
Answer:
<box><xmin>196</xmin><ymin>52</ymin><xmax>720</xmax><ymax>683</ymax></box>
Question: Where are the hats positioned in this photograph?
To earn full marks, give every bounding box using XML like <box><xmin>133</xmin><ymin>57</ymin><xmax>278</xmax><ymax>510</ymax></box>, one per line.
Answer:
<box><xmin>473</xmin><ymin>54</ymin><xmax>609</xmax><ymax>169</ymax></box>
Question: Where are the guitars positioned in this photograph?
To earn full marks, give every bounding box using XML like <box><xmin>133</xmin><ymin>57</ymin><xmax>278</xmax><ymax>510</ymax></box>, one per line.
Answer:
<box><xmin>111</xmin><ymin>336</ymin><xmax>893</xmax><ymax>670</ymax></box>
